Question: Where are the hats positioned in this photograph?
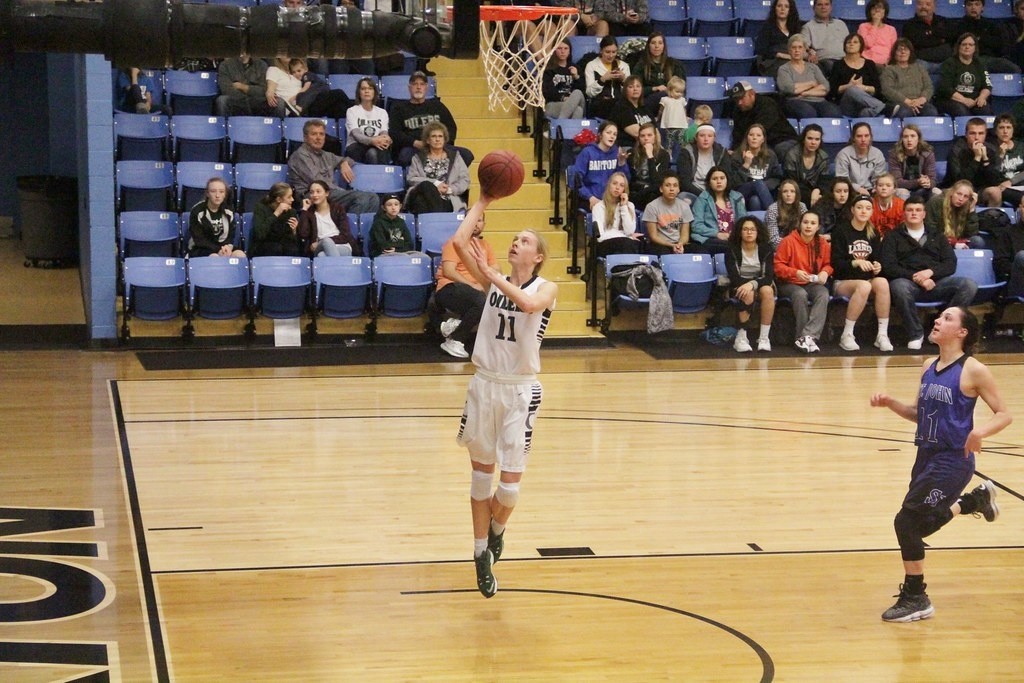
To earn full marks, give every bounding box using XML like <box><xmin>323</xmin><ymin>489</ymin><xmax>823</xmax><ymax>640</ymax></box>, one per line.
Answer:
<box><xmin>383</xmin><ymin>193</ymin><xmax>401</xmax><ymax>205</ymax></box>
<box><xmin>730</xmin><ymin>80</ymin><xmax>752</xmax><ymax>98</ymax></box>
<box><xmin>409</xmin><ymin>71</ymin><xmax>428</xmax><ymax>83</ymax></box>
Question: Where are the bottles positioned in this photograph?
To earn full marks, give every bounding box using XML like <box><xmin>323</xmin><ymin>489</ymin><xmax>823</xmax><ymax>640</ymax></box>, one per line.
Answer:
<box><xmin>806</xmin><ymin>273</ymin><xmax>818</xmax><ymax>283</ymax></box>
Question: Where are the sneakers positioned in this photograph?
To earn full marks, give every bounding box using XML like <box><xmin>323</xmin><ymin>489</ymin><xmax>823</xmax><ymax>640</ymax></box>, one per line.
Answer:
<box><xmin>882</xmin><ymin>583</ymin><xmax>935</xmax><ymax>623</ymax></box>
<box><xmin>756</xmin><ymin>337</ymin><xmax>771</xmax><ymax>351</ymax></box>
<box><xmin>908</xmin><ymin>334</ymin><xmax>924</xmax><ymax>350</ymax></box>
<box><xmin>487</xmin><ymin>517</ymin><xmax>505</xmax><ymax>565</ymax></box>
<box><xmin>440</xmin><ymin>317</ymin><xmax>462</xmax><ymax>338</ymax></box>
<box><xmin>733</xmin><ymin>335</ymin><xmax>753</xmax><ymax>353</ymax></box>
<box><xmin>874</xmin><ymin>334</ymin><xmax>893</xmax><ymax>351</ymax></box>
<box><xmin>474</xmin><ymin>548</ymin><xmax>497</xmax><ymax>598</ymax></box>
<box><xmin>794</xmin><ymin>335</ymin><xmax>820</xmax><ymax>354</ymax></box>
<box><xmin>440</xmin><ymin>337</ymin><xmax>469</xmax><ymax>359</ymax></box>
<box><xmin>839</xmin><ymin>332</ymin><xmax>860</xmax><ymax>351</ymax></box>
<box><xmin>969</xmin><ymin>481</ymin><xmax>999</xmax><ymax>522</ymax></box>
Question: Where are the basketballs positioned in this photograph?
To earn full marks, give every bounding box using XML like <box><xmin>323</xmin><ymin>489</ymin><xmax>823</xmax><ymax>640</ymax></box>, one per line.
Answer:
<box><xmin>478</xmin><ymin>149</ymin><xmax>525</xmax><ymax>199</ymax></box>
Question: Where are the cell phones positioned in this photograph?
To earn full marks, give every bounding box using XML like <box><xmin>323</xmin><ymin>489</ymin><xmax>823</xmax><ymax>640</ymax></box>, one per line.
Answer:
<box><xmin>613</xmin><ymin>68</ymin><xmax>620</xmax><ymax>71</ymax></box>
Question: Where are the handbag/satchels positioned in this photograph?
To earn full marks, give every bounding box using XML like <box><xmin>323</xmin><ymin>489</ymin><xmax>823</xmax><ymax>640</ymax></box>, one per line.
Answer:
<box><xmin>610</xmin><ymin>261</ymin><xmax>668</xmax><ymax>297</ymax></box>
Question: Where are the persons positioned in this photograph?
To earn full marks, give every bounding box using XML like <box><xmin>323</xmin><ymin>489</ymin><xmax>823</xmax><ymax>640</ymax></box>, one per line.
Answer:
<box><xmin>434</xmin><ymin>208</ymin><xmax>500</xmax><ymax>359</ymax></box>
<box><xmin>117</xmin><ymin>52</ymin><xmax>474</xmax><ymax>256</ymax></box>
<box><xmin>453</xmin><ymin>184</ymin><xmax>558</xmax><ymax>598</ymax></box>
<box><xmin>870</xmin><ymin>307</ymin><xmax>1013</xmax><ymax>623</ymax></box>
<box><xmin>515</xmin><ymin>0</ymin><xmax>1024</xmax><ymax>352</ymax></box>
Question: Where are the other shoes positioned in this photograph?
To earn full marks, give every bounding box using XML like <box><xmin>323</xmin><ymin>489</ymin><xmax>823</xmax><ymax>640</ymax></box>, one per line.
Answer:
<box><xmin>882</xmin><ymin>102</ymin><xmax>900</xmax><ymax>118</ymax></box>
<box><xmin>284</xmin><ymin>98</ymin><xmax>300</xmax><ymax>117</ymax></box>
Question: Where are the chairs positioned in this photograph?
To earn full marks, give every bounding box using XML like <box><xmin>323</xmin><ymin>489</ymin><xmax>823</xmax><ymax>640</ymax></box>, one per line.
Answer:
<box><xmin>495</xmin><ymin>0</ymin><xmax>1024</xmax><ymax>341</ymax></box>
<box><xmin>111</xmin><ymin>0</ymin><xmax>469</xmax><ymax>346</ymax></box>
